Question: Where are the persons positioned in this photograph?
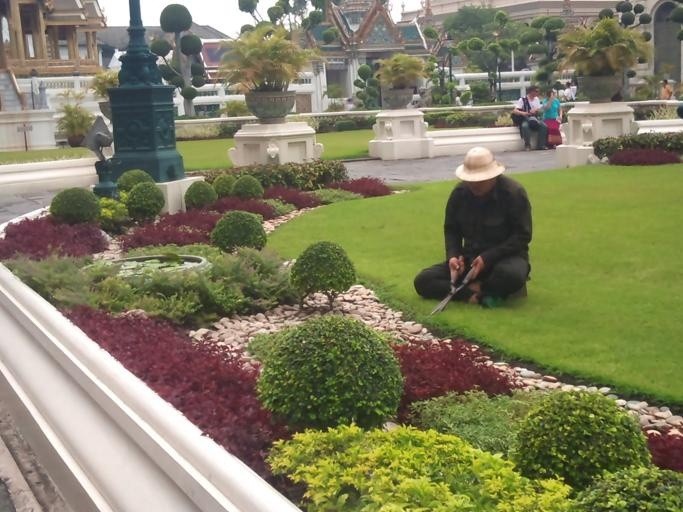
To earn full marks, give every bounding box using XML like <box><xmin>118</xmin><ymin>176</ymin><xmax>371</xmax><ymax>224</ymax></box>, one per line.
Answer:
<box><xmin>343</xmin><ymin>98</ymin><xmax>356</xmax><ymax>110</ymax></box>
<box><xmin>563</xmin><ymin>82</ymin><xmax>575</xmax><ymax>101</ymax></box>
<box><xmin>511</xmin><ymin>85</ymin><xmax>549</xmax><ymax>151</ymax></box>
<box><xmin>416</xmin><ymin>92</ymin><xmax>427</xmax><ymax>107</ymax></box>
<box><xmin>658</xmin><ymin>79</ymin><xmax>673</xmax><ymax>99</ymax></box>
<box><xmin>456</xmin><ymin>95</ymin><xmax>462</xmax><ymax>105</ymax></box>
<box><xmin>541</xmin><ymin>90</ymin><xmax>563</xmax><ymax>150</ymax></box>
<box><xmin>413</xmin><ymin>145</ymin><xmax>534</xmax><ymax>308</ymax></box>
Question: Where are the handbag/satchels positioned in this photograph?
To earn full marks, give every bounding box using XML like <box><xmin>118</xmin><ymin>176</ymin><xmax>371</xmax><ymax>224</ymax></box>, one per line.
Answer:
<box><xmin>511</xmin><ymin>109</ymin><xmax>525</xmax><ymax>125</ymax></box>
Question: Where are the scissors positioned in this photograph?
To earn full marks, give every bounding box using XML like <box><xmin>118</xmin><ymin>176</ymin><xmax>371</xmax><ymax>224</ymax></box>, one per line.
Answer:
<box><xmin>430</xmin><ymin>255</ymin><xmax>476</xmax><ymax>316</ymax></box>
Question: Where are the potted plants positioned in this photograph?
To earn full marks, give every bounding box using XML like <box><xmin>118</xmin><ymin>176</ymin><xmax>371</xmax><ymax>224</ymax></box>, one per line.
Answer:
<box><xmin>371</xmin><ymin>53</ymin><xmax>432</xmax><ymax>109</ymax></box>
<box><xmin>213</xmin><ymin>22</ymin><xmax>328</xmax><ymax>124</ymax></box>
<box><xmin>556</xmin><ymin>16</ymin><xmax>657</xmax><ymax>103</ymax></box>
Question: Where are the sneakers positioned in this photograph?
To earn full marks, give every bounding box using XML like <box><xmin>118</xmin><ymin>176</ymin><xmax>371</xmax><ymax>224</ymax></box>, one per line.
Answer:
<box><xmin>525</xmin><ymin>144</ymin><xmax>556</xmax><ymax>150</ymax></box>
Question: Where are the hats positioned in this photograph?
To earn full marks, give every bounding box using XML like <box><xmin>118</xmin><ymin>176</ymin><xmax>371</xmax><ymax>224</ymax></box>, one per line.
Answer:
<box><xmin>455</xmin><ymin>147</ymin><xmax>503</xmax><ymax>182</ymax></box>
